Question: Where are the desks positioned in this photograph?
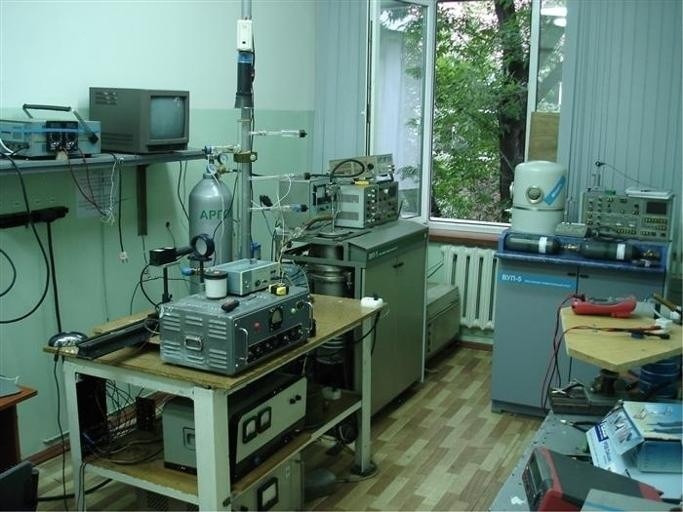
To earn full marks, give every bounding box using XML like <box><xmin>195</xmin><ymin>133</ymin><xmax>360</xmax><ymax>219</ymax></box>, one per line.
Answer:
<box><xmin>483</xmin><ymin>393</ymin><xmax>683</xmax><ymax>512</ymax></box>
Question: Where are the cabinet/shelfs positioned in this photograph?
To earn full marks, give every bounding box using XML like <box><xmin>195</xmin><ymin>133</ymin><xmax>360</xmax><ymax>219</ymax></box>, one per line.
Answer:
<box><xmin>41</xmin><ymin>284</ymin><xmax>387</xmax><ymax>511</ymax></box>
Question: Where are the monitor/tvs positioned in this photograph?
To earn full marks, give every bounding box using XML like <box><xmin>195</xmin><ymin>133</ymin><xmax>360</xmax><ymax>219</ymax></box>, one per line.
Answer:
<box><xmin>89</xmin><ymin>87</ymin><xmax>189</xmax><ymax>154</ymax></box>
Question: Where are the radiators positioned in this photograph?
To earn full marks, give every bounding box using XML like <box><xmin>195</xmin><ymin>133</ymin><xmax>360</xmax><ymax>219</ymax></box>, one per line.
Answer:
<box><xmin>439</xmin><ymin>244</ymin><xmax>496</xmax><ymax>332</ymax></box>
